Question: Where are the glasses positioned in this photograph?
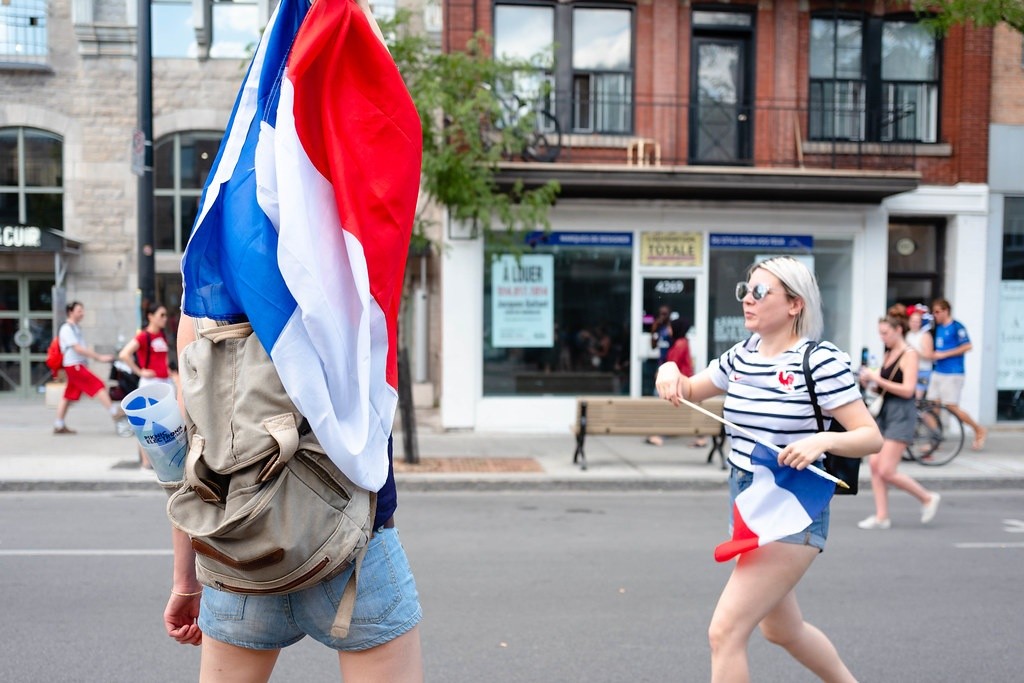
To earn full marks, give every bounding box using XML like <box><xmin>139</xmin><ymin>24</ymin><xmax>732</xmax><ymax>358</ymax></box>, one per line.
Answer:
<box><xmin>735</xmin><ymin>281</ymin><xmax>794</xmax><ymax>302</ymax></box>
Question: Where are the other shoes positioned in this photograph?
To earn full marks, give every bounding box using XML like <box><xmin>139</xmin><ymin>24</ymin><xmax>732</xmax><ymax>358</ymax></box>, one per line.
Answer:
<box><xmin>921</xmin><ymin>493</ymin><xmax>940</xmax><ymax>524</ymax></box>
<box><xmin>54</xmin><ymin>428</ymin><xmax>75</xmax><ymax>435</ymax></box>
<box><xmin>858</xmin><ymin>516</ymin><xmax>891</xmax><ymax>530</ymax></box>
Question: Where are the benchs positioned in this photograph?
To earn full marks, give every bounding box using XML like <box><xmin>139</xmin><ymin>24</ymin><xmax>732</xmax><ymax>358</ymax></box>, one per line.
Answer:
<box><xmin>480</xmin><ymin>130</ymin><xmax>661</xmax><ymax>168</ymax></box>
<box><xmin>570</xmin><ymin>398</ymin><xmax>729</xmax><ymax>471</ymax></box>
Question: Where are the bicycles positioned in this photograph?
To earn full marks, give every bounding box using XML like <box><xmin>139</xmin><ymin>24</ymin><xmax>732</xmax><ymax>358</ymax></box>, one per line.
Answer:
<box><xmin>457</xmin><ymin>80</ymin><xmax>562</xmax><ymax>163</ymax></box>
<box><xmin>851</xmin><ymin>372</ymin><xmax>965</xmax><ymax>467</ymax></box>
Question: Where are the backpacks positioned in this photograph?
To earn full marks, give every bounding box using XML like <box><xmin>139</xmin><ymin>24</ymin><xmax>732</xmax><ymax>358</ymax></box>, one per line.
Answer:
<box><xmin>157</xmin><ymin>313</ymin><xmax>375</xmax><ymax>597</ymax></box>
<box><xmin>119</xmin><ymin>331</ymin><xmax>150</xmax><ymax>393</ymax></box>
<box><xmin>46</xmin><ymin>322</ymin><xmax>72</xmax><ymax>370</ymax></box>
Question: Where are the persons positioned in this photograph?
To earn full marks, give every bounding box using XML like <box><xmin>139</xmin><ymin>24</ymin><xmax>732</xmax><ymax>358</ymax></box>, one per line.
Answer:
<box><xmin>891</xmin><ymin>300</ymin><xmax>989</xmax><ymax>460</ymax></box>
<box><xmin>655</xmin><ymin>255</ymin><xmax>883</xmax><ymax>683</ymax></box>
<box><xmin>645</xmin><ymin>305</ymin><xmax>707</xmax><ymax>448</ymax></box>
<box><xmin>503</xmin><ymin>322</ymin><xmax>621</xmax><ymax>371</ymax></box>
<box><xmin>119</xmin><ymin>304</ymin><xmax>179</xmax><ymax>468</ymax></box>
<box><xmin>52</xmin><ymin>300</ymin><xmax>127</xmax><ymax>432</ymax></box>
<box><xmin>163</xmin><ymin>312</ymin><xmax>422</xmax><ymax>683</ymax></box>
<box><xmin>858</xmin><ymin>314</ymin><xmax>941</xmax><ymax>530</ymax></box>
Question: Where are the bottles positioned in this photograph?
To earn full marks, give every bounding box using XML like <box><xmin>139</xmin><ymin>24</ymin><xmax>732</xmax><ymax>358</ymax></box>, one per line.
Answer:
<box><xmin>870</xmin><ymin>354</ymin><xmax>878</xmax><ymax>388</ymax></box>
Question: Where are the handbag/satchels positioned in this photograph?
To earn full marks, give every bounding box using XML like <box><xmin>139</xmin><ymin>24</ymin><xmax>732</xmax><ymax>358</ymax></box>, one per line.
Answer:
<box><xmin>863</xmin><ymin>390</ymin><xmax>884</xmax><ymax>418</ymax></box>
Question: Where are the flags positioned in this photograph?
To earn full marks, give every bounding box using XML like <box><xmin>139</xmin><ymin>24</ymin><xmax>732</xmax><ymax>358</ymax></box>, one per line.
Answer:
<box><xmin>182</xmin><ymin>0</ymin><xmax>421</xmax><ymax>493</ymax></box>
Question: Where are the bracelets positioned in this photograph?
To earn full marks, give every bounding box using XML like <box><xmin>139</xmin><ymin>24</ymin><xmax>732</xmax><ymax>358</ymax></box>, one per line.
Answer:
<box><xmin>170</xmin><ymin>590</ymin><xmax>202</xmax><ymax>596</ymax></box>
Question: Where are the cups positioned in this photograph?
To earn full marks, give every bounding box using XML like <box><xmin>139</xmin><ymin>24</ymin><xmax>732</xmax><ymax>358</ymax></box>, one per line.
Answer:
<box><xmin>121</xmin><ymin>383</ymin><xmax>188</xmax><ymax>482</ymax></box>
